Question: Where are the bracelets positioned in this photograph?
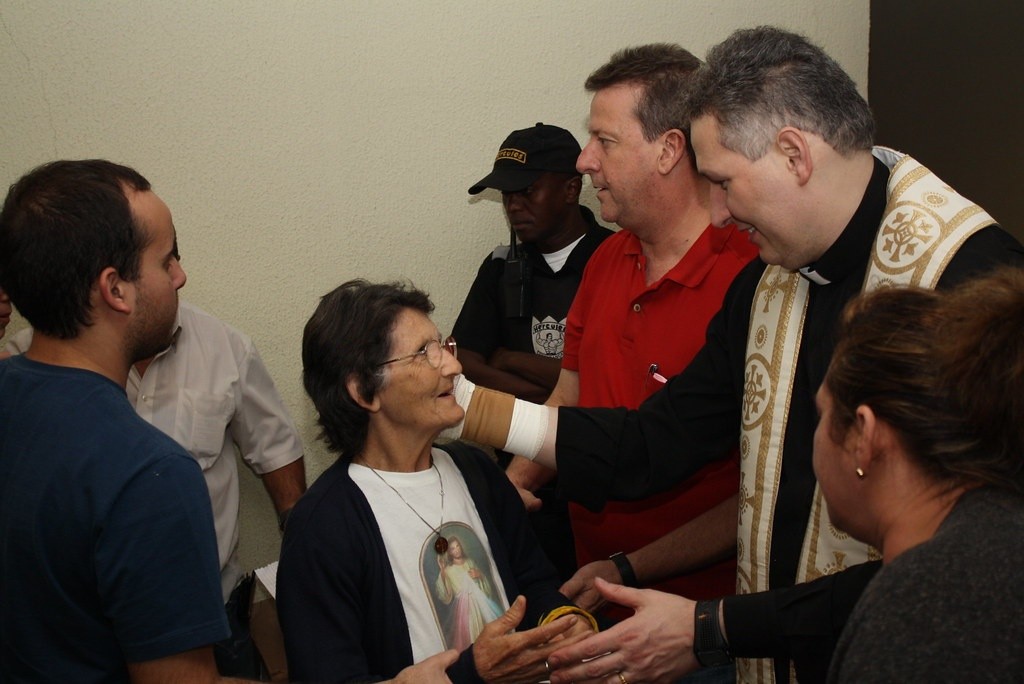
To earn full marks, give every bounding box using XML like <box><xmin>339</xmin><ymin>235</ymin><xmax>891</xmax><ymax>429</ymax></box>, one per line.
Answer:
<box><xmin>538</xmin><ymin>604</ymin><xmax>600</xmax><ymax>643</ymax></box>
<box><xmin>608</xmin><ymin>551</ymin><xmax>639</xmax><ymax>587</ymax></box>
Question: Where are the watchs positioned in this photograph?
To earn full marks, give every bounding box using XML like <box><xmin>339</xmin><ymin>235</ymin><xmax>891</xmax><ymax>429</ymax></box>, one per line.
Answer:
<box><xmin>695</xmin><ymin>598</ymin><xmax>733</xmax><ymax>668</ymax></box>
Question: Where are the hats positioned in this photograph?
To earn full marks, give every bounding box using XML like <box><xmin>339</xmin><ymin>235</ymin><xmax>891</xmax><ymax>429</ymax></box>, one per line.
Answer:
<box><xmin>468</xmin><ymin>122</ymin><xmax>583</xmax><ymax>195</ymax></box>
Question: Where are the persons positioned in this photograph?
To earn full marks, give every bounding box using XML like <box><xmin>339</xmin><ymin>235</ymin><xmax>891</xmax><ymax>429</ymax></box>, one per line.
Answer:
<box><xmin>433</xmin><ymin>24</ymin><xmax>1024</xmax><ymax>684</ymax></box>
<box><xmin>451</xmin><ymin>120</ymin><xmax>616</xmax><ymax>593</ymax></box>
<box><xmin>276</xmin><ymin>279</ymin><xmax>603</xmax><ymax>684</ymax></box>
<box><xmin>503</xmin><ymin>45</ymin><xmax>762</xmax><ymax>628</ymax></box>
<box><xmin>813</xmin><ymin>269</ymin><xmax>1024</xmax><ymax>684</ymax></box>
<box><xmin>0</xmin><ymin>158</ymin><xmax>306</xmax><ymax>684</ymax></box>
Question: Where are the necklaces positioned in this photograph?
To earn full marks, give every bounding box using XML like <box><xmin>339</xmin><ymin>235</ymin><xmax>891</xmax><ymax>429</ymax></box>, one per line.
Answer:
<box><xmin>359</xmin><ymin>454</ymin><xmax>449</xmax><ymax>553</ymax></box>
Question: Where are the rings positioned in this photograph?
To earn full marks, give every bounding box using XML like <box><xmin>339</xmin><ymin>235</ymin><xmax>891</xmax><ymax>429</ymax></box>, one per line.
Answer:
<box><xmin>545</xmin><ymin>662</ymin><xmax>550</xmax><ymax>674</ymax></box>
<box><xmin>618</xmin><ymin>671</ymin><xmax>627</xmax><ymax>684</ymax></box>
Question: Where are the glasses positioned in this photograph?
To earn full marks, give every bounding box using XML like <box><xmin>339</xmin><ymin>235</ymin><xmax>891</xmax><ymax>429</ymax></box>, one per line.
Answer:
<box><xmin>372</xmin><ymin>338</ymin><xmax>458</xmax><ymax>369</ymax></box>
